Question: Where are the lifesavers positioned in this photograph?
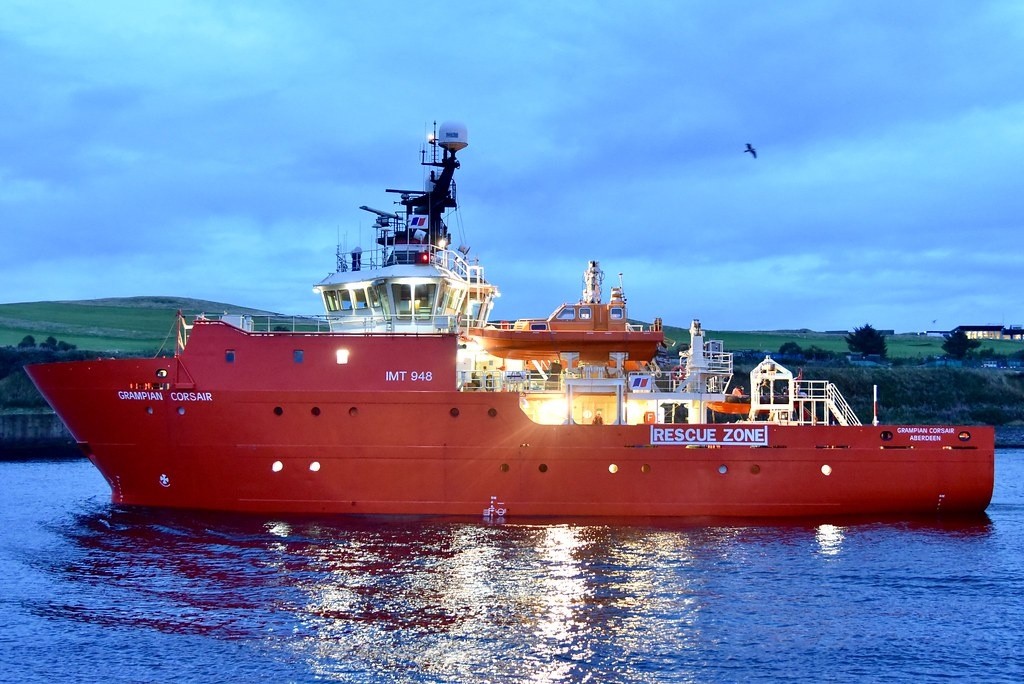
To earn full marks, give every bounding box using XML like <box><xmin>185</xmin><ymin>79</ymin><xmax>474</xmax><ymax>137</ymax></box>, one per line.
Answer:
<box><xmin>672</xmin><ymin>365</ymin><xmax>686</xmax><ymax>381</ymax></box>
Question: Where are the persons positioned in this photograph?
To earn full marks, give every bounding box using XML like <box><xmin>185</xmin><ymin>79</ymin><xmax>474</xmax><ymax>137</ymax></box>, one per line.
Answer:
<box><xmin>593</xmin><ymin>410</ymin><xmax>602</xmax><ymax>425</ymax></box>
<box><xmin>732</xmin><ymin>385</ymin><xmax>744</xmax><ymax>395</ymax></box>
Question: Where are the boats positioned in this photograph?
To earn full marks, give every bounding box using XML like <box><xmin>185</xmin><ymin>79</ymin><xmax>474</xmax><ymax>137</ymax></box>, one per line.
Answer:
<box><xmin>23</xmin><ymin>124</ymin><xmax>999</xmax><ymax>528</ymax></box>
<box><xmin>706</xmin><ymin>400</ymin><xmax>753</xmax><ymax>415</ymax></box>
